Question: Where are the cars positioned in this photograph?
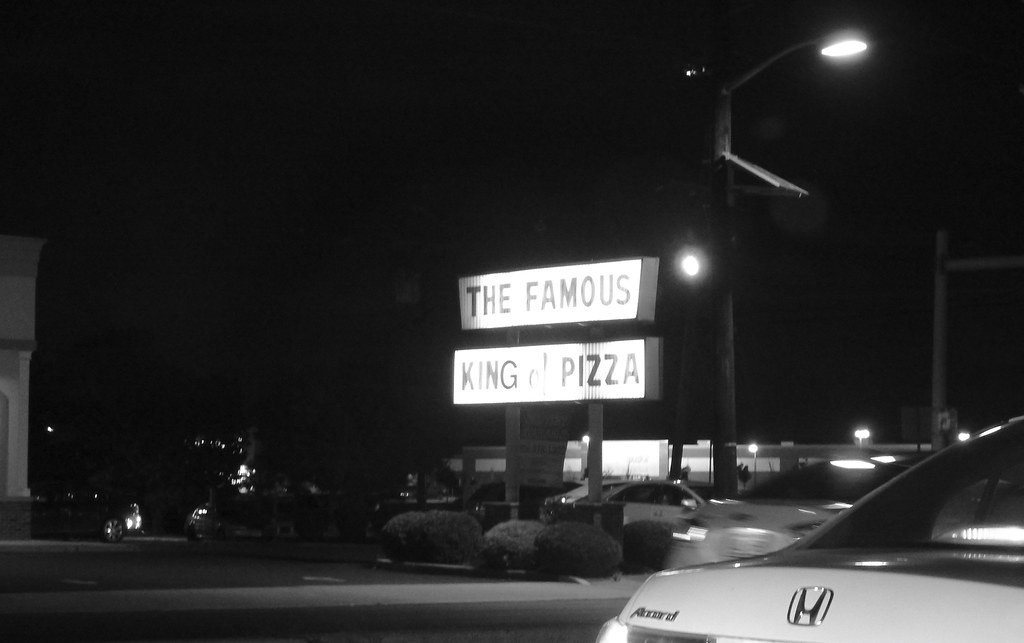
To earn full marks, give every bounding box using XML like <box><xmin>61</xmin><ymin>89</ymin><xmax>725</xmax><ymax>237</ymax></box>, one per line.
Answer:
<box><xmin>543</xmin><ymin>479</ymin><xmax>635</xmax><ymax>507</ymax></box>
<box><xmin>471</xmin><ymin>480</ymin><xmax>583</xmax><ymax>526</ymax></box>
<box><xmin>661</xmin><ymin>454</ymin><xmax>914</xmax><ymax>565</ymax></box>
<box><xmin>30</xmin><ymin>482</ymin><xmax>143</xmax><ymax>544</ymax></box>
<box><xmin>594</xmin><ymin>417</ymin><xmax>1024</xmax><ymax>643</ymax></box>
<box><xmin>600</xmin><ymin>480</ymin><xmax>746</xmax><ymax>528</ymax></box>
<box><xmin>184</xmin><ymin>501</ymin><xmax>278</xmax><ymax>544</ymax></box>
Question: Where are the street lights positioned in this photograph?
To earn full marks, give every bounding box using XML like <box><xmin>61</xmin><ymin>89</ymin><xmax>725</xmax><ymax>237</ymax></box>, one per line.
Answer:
<box><xmin>716</xmin><ymin>23</ymin><xmax>871</xmax><ymax>504</ymax></box>
